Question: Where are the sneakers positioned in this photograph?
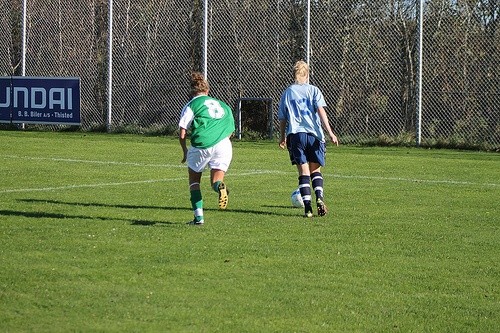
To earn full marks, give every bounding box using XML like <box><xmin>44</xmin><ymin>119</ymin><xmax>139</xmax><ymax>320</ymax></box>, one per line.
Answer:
<box><xmin>217</xmin><ymin>182</ymin><xmax>229</xmax><ymax>210</ymax></box>
<box><xmin>305</xmin><ymin>206</ymin><xmax>313</xmax><ymax>218</ymax></box>
<box><xmin>316</xmin><ymin>197</ymin><xmax>329</xmax><ymax>217</ymax></box>
<box><xmin>186</xmin><ymin>220</ymin><xmax>205</xmax><ymax>226</ymax></box>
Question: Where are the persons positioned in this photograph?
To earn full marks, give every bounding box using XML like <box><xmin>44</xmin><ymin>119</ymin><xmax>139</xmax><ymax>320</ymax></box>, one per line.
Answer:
<box><xmin>278</xmin><ymin>61</ymin><xmax>339</xmax><ymax>216</ymax></box>
<box><xmin>179</xmin><ymin>73</ymin><xmax>235</xmax><ymax>224</ymax></box>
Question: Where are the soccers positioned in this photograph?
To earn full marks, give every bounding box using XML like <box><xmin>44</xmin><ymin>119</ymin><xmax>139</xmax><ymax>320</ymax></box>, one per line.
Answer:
<box><xmin>291</xmin><ymin>188</ymin><xmax>305</xmax><ymax>208</ymax></box>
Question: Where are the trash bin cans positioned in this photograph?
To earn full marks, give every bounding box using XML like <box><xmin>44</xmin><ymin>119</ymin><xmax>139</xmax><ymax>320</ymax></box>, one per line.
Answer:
<box><xmin>237</xmin><ymin>97</ymin><xmax>273</xmax><ymax>141</ymax></box>
